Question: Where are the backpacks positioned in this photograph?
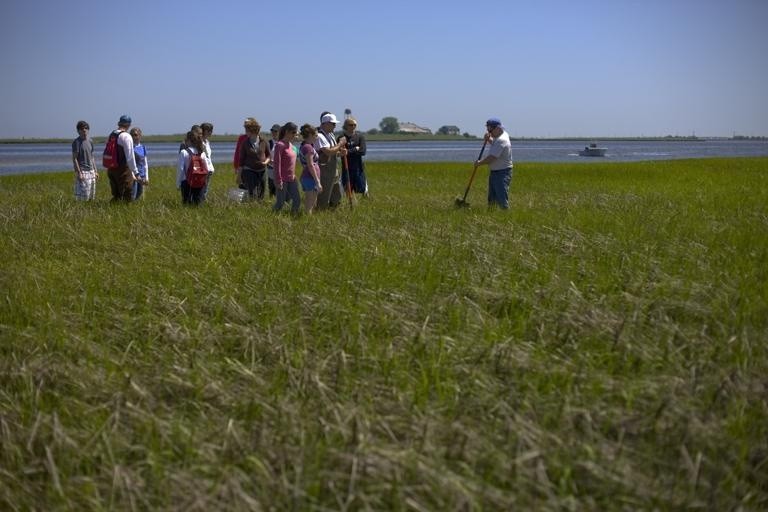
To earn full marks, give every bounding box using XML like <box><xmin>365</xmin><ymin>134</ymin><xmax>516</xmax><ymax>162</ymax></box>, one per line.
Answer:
<box><xmin>184</xmin><ymin>147</ymin><xmax>207</xmax><ymax>187</ymax></box>
<box><xmin>104</xmin><ymin>131</ymin><xmax>125</xmax><ymax>169</ymax></box>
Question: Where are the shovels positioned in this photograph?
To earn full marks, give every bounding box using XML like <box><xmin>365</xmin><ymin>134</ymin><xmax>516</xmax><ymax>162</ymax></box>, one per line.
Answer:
<box><xmin>455</xmin><ymin>135</ymin><xmax>490</xmax><ymax>207</ymax></box>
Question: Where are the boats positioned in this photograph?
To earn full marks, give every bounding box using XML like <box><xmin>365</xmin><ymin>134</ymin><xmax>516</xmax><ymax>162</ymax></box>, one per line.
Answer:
<box><xmin>577</xmin><ymin>143</ymin><xmax>608</xmax><ymax>156</ymax></box>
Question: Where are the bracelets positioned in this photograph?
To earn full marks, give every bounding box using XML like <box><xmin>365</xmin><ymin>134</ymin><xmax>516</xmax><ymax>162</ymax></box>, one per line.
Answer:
<box><xmin>478</xmin><ymin>160</ymin><xmax>481</xmax><ymax>166</ymax></box>
<box><xmin>135</xmin><ymin>173</ymin><xmax>140</xmax><ymax>177</ymax></box>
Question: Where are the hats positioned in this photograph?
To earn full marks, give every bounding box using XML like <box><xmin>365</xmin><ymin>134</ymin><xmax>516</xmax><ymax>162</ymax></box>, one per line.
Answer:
<box><xmin>120</xmin><ymin>116</ymin><xmax>131</xmax><ymax>122</ymax></box>
<box><xmin>487</xmin><ymin>118</ymin><xmax>506</xmax><ymax>129</ymax></box>
<box><xmin>322</xmin><ymin>114</ymin><xmax>340</xmax><ymax>123</ymax></box>
<box><xmin>270</xmin><ymin>124</ymin><xmax>280</xmax><ymax>131</ymax></box>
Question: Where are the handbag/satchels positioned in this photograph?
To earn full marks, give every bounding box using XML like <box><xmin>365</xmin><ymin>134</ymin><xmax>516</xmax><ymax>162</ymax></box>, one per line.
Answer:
<box><xmin>228</xmin><ymin>187</ymin><xmax>248</xmax><ymax>202</ymax></box>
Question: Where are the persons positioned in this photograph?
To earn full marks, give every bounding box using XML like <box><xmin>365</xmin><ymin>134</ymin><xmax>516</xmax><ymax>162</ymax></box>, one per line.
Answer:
<box><xmin>129</xmin><ymin>127</ymin><xmax>148</xmax><ymax>202</ymax></box>
<box><xmin>72</xmin><ymin>120</ymin><xmax>99</xmax><ymax>202</ymax></box>
<box><xmin>103</xmin><ymin>114</ymin><xmax>143</xmax><ymax>203</ymax></box>
<box><xmin>176</xmin><ymin>112</ymin><xmax>369</xmax><ymax>215</ymax></box>
<box><xmin>473</xmin><ymin>117</ymin><xmax>513</xmax><ymax>210</ymax></box>
